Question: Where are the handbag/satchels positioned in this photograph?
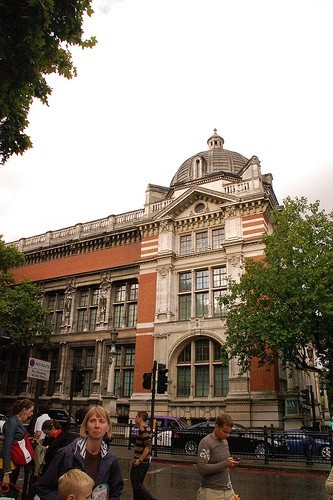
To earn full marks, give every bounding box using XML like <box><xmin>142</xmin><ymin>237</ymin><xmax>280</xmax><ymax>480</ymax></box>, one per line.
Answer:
<box><xmin>10</xmin><ymin>432</ymin><xmax>35</xmax><ymax>466</ymax></box>
<box><xmin>0</xmin><ymin>481</ymin><xmax>21</xmax><ymax>500</ymax></box>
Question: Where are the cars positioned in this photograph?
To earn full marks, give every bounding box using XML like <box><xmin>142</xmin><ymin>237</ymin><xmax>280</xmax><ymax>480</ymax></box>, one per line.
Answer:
<box><xmin>49</xmin><ymin>409</ymin><xmax>75</xmax><ymax>430</ymax></box>
<box><xmin>174</xmin><ymin>420</ymin><xmax>287</xmax><ymax>463</ymax></box>
<box><xmin>129</xmin><ymin>415</ymin><xmax>189</xmax><ymax>447</ymax></box>
<box><xmin>270</xmin><ymin>429</ymin><xmax>331</xmax><ymax>460</ymax></box>
<box><xmin>0</xmin><ymin>414</ymin><xmax>8</xmax><ymax>433</ymax></box>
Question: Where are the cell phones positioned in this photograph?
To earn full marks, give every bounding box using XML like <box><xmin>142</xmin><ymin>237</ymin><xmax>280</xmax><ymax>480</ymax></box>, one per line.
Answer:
<box><xmin>234</xmin><ymin>457</ymin><xmax>242</xmax><ymax>461</ymax></box>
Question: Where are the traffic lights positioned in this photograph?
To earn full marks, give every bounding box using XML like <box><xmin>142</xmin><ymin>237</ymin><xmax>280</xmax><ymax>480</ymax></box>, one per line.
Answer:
<box><xmin>143</xmin><ymin>373</ymin><xmax>152</xmax><ymax>390</ymax></box>
<box><xmin>157</xmin><ymin>363</ymin><xmax>169</xmax><ymax>394</ymax></box>
<box><xmin>301</xmin><ymin>389</ymin><xmax>310</xmax><ymax>405</ymax></box>
<box><xmin>74</xmin><ymin>365</ymin><xmax>86</xmax><ymax>392</ymax></box>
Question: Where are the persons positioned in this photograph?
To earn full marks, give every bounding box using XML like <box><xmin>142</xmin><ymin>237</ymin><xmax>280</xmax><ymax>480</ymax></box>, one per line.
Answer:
<box><xmin>325</xmin><ymin>467</ymin><xmax>333</xmax><ymax>500</ymax></box>
<box><xmin>196</xmin><ymin>414</ymin><xmax>240</xmax><ymax>500</ymax></box>
<box><xmin>59</xmin><ymin>469</ymin><xmax>95</xmax><ymax>500</ymax></box>
<box><xmin>29</xmin><ymin>404</ymin><xmax>85</xmax><ymax>475</ymax></box>
<box><xmin>0</xmin><ymin>399</ymin><xmax>41</xmax><ymax>500</ymax></box>
<box><xmin>37</xmin><ymin>406</ymin><xmax>124</xmax><ymax>500</ymax></box>
<box><xmin>130</xmin><ymin>411</ymin><xmax>157</xmax><ymax>500</ymax></box>
